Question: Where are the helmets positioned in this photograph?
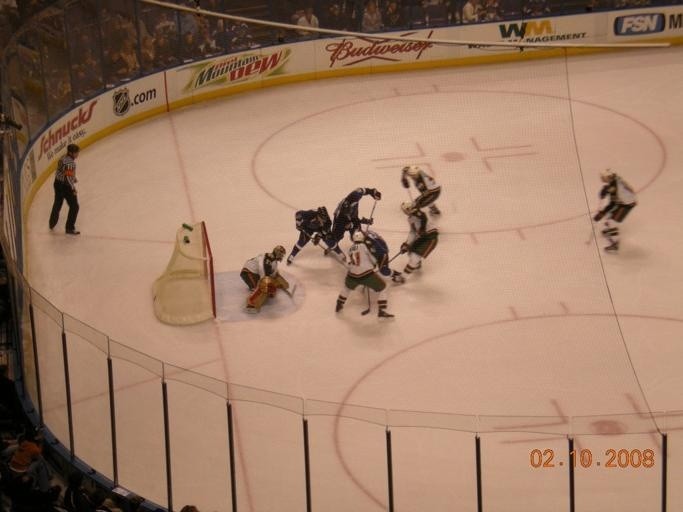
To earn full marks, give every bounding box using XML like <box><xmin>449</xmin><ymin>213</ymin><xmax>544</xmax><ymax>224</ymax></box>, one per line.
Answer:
<box><xmin>274</xmin><ymin>245</ymin><xmax>286</xmax><ymax>257</ymax></box>
<box><xmin>317</xmin><ymin>207</ymin><xmax>327</xmax><ymax>220</ymax></box>
<box><xmin>401</xmin><ymin>201</ymin><xmax>414</xmax><ymax>216</ymax></box>
<box><xmin>342</xmin><ymin>200</ymin><xmax>351</xmax><ymax>214</ymax></box>
<box><xmin>352</xmin><ymin>231</ymin><xmax>364</xmax><ymax>242</ymax></box>
<box><xmin>600</xmin><ymin>167</ymin><xmax>614</xmax><ymax>177</ymax></box>
<box><xmin>68</xmin><ymin>144</ymin><xmax>80</xmax><ymax>151</ymax></box>
<box><xmin>407</xmin><ymin>168</ymin><xmax>419</xmax><ymax>180</ymax></box>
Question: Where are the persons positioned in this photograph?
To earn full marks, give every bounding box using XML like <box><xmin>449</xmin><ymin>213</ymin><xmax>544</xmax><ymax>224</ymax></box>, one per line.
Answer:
<box><xmin>0</xmin><ymin>0</ymin><xmax>641</xmax><ymax>122</ymax></box>
<box><xmin>1</xmin><ymin>424</ymin><xmax>198</xmax><ymax>510</ymax></box>
<box><xmin>240</xmin><ymin>163</ymin><xmax>442</xmax><ymax>321</ymax></box>
<box><xmin>44</xmin><ymin>144</ymin><xmax>83</xmax><ymax>235</ymax></box>
<box><xmin>593</xmin><ymin>168</ymin><xmax>637</xmax><ymax>252</ymax></box>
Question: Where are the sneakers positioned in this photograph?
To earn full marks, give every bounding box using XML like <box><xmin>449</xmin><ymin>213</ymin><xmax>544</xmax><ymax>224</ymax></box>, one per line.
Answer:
<box><xmin>391</xmin><ymin>276</ymin><xmax>408</xmax><ymax>284</ymax></box>
<box><xmin>287</xmin><ymin>254</ymin><xmax>295</xmax><ymax>264</ymax></box>
<box><xmin>605</xmin><ymin>242</ymin><xmax>618</xmax><ymax>250</ymax></box>
<box><xmin>429</xmin><ymin>205</ymin><xmax>439</xmax><ymax>214</ymax></box>
<box><xmin>65</xmin><ymin>227</ymin><xmax>80</xmax><ymax>235</ymax></box>
<box><xmin>337</xmin><ymin>250</ymin><xmax>345</xmax><ymax>260</ymax></box>
<box><xmin>378</xmin><ymin>307</ymin><xmax>394</xmax><ymax>318</ymax></box>
<box><xmin>335</xmin><ymin>301</ymin><xmax>344</xmax><ymax>312</ymax></box>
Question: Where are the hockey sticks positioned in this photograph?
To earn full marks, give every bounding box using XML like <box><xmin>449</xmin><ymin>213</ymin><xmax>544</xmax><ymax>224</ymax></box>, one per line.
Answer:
<box><xmin>360</xmin><ymin>288</ymin><xmax>371</xmax><ymax>315</ymax></box>
<box><xmin>281</xmin><ymin>284</ymin><xmax>296</xmax><ymax>298</ymax></box>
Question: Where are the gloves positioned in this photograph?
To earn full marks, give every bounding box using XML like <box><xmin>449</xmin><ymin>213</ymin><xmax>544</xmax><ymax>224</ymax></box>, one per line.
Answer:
<box><xmin>401</xmin><ymin>242</ymin><xmax>408</xmax><ymax>255</ymax></box>
<box><xmin>401</xmin><ymin>178</ymin><xmax>409</xmax><ymax>188</ymax></box>
<box><xmin>296</xmin><ymin>218</ymin><xmax>304</xmax><ymax>232</ymax></box>
<box><xmin>366</xmin><ymin>189</ymin><xmax>381</xmax><ymax>199</ymax></box>
<box><xmin>314</xmin><ymin>234</ymin><xmax>320</xmax><ymax>246</ymax></box>
<box><xmin>366</xmin><ymin>217</ymin><xmax>374</xmax><ymax>225</ymax></box>
